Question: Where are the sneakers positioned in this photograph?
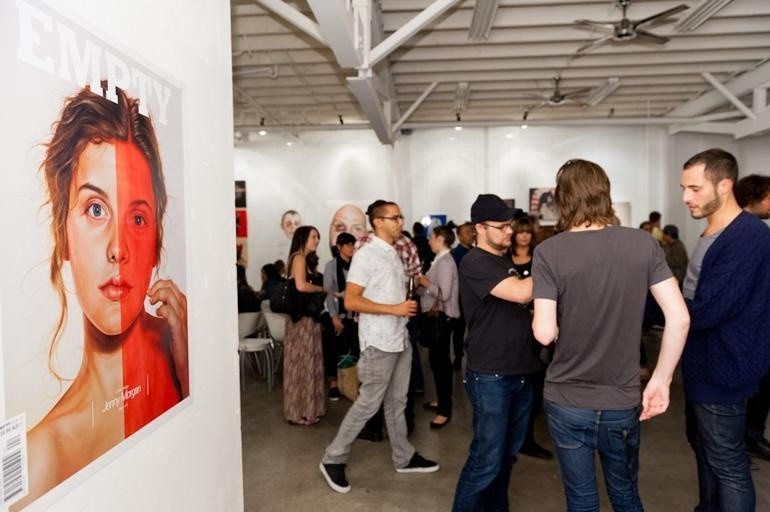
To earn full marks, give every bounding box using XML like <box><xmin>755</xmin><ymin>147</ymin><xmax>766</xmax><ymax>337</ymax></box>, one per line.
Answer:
<box><xmin>319</xmin><ymin>460</ymin><xmax>351</xmax><ymax>493</ymax></box>
<box><xmin>326</xmin><ymin>387</ymin><xmax>341</xmax><ymax>401</ymax></box>
<box><xmin>396</xmin><ymin>451</ymin><xmax>439</xmax><ymax>475</ymax></box>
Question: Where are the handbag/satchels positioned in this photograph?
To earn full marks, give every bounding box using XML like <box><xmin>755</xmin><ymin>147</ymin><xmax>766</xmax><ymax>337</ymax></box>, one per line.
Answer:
<box><xmin>300</xmin><ymin>283</ymin><xmax>301</xmax><ymax>284</ymax></box>
<box><xmin>419</xmin><ymin>287</ymin><xmax>447</xmax><ymax>348</ymax></box>
<box><xmin>271</xmin><ymin>252</ymin><xmax>326</xmax><ymax>317</ymax></box>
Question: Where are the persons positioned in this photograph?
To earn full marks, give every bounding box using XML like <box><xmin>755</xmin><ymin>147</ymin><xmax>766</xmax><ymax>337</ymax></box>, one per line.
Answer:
<box><xmin>8</xmin><ymin>85</ymin><xmax>189</xmax><ymax>512</ymax></box>
<box><xmin>680</xmin><ymin>147</ymin><xmax>770</xmax><ymax>512</ymax></box>
<box><xmin>510</xmin><ymin>219</ymin><xmax>555</xmax><ymax>461</ymax></box>
<box><xmin>419</xmin><ymin>220</ymin><xmax>461</xmax><ymax>430</ymax></box>
<box><xmin>533</xmin><ymin>158</ymin><xmax>691</xmax><ymax>507</ymax></box>
<box><xmin>648</xmin><ymin>211</ymin><xmax>661</xmax><ymax>243</ymax></box>
<box><xmin>662</xmin><ymin>224</ymin><xmax>689</xmax><ymax>284</ymax></box>
<box><xmin>282</xmin><ymin>226</ymin><xmax>324</xmax><ymax>426</ymax></box>
<box><xmin>452</xmin><ymin>194</ymin><xmax>534</xmax><ymax>511</ymax></box>
<box><xmin>319</xmin><ymin>199</ymin><xmax>441</xmax><ymax>494</ymax></box>
<box><xmin>237</xmin><ymin>264</ymin><xmax>262</xmax><ymax>382</ymax></box>
<box><xmin>269</xmin><ymin>211</ymin><xmax>302</xmax><ymax>266</ymax></box>
<box><xmin>330</xmin><ymin>204</ymin><xmax>367</xmax><ymax>259</ymax></box>
<box><xmin>450</xmin><ymin>224</ymin><xmax>475</xmax><ymax>270</ymax></box>
<box><xmin>639</xmin><ymin>222</ymin><xmax>654</xmax><ymax>233</ymax></box>
<box><xmin>735</xmin><ymin>173</ymin><xmax>770</xmax><ymax>220</ymax></box>
<box><xmin>354</xmin><ymin>231</ymin><xmax>421</xmax><ymax>442</ymax></box>
<box><xmin>323</xmin><ymin>232</ymin><xmax>354</xmax><ymax>401</ymax></box>
<box><xmin>410</xmin><ymin>221</ymin><xmax>435</xmax><ymax>276</ymax></box>
<box><xmin>260</xmin><ymin>260</ymin><xmax>285</xmax><ymax>300</ymax></box>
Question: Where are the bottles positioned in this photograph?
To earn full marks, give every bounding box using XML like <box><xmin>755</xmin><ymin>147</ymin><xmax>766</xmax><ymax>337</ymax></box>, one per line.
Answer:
<box><xmin>407</xmin><ymin>276</ymin><xmax>416</xmax><ymax>301</ymax></box>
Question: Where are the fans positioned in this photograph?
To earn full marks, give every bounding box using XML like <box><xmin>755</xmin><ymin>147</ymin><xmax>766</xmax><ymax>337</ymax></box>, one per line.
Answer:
<box><xmin>572</xmin><ymin>0</ymin><xmax>689</xmax><ymax>56</ymax></box>
<box><xmin>522</xmin><ymin>75</ymin><xmax>600</xmax><ymax>110</ymax></box>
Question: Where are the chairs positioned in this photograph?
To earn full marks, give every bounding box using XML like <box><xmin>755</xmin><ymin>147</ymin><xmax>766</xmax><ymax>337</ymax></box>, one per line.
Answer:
<box><xmin>262</xmin><ymin>312</ymin><xmax>290</xmax><ymax>376</ymax></box>
<box><xmin>239</xmin><ymin>312</ymin><xmax>264</xmax><ymax>378</ymax></box>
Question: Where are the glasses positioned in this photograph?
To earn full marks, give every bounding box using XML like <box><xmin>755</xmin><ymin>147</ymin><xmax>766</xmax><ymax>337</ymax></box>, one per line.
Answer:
<box><xmin>483</xmin><ymin>220</ymin><xmax>515</xmax><ymax>230</ymax></box>
<box><xmin>376</xmin><ymin>215</ymin><xmax>403</xmax><ymax>221</ymax></box>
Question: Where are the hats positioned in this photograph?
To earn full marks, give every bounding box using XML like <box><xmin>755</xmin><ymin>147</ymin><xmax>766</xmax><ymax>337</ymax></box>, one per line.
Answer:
<box><xmin>471</xmin><ymin>194</ymin><xmax>517</xmax><ymax>224</ymax></box>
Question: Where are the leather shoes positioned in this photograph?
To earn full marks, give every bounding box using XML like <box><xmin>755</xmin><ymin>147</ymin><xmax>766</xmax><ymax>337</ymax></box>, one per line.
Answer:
<box><xmin>746</xmin><ymin>432</ymin><xmax>770</xmax><ymax>460</ymax></box>
<box><xmin>428</xmin><ymin>412</ymin><xmax>449</xmax><ymax>429</ymax></box>
<box><xmin>423</xmin><ymin>400</ymin><xmax>438</xmax><ymax>411</ymax></box>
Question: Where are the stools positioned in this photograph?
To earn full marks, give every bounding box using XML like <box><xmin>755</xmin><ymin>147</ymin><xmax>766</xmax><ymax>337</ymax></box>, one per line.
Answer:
<box><xmin>239</xmin><ymin>338</ymin><xmax>275</xmax><ymax>392</ymax></box>
